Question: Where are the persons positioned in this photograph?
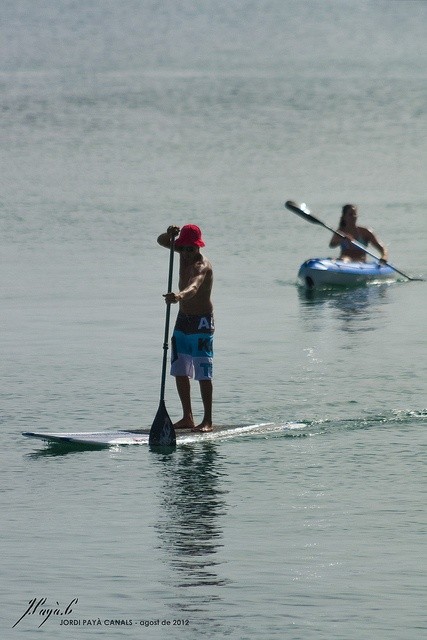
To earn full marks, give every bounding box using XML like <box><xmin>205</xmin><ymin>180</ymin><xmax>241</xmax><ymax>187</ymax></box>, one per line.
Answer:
<box><xmin>328</xmin><ymin>203</ymin><xmax>389</xmax><ymax>266</ymax></box>
<box><xmin>156</xmin><ymin>222</ymin><xmax>216</xmax><ymax>435</ymax></box>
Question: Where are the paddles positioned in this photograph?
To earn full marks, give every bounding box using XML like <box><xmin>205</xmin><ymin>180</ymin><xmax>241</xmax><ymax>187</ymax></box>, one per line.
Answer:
<box><xmin>285</xmin><ymin>200</ymin><xmax>413</xmax><ymax>281</ymax></box>
<box><xmin>149</xmin><ymin>224</ymin><xmax>176</xmax><ymax>452</ymax></box>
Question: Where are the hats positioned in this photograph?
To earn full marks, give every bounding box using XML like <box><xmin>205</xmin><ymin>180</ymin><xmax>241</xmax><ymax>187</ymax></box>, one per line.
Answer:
<box><xmin>174</xmin><ymin>225</ymin><xmax>205</xmax><ymax>247</ymax></box>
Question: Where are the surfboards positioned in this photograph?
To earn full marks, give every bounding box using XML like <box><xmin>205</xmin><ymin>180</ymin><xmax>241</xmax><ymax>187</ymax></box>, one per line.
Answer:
<box><xmin>21</xmin><ymin>422</ymin><xmax>275</xmax><ymax>446</ymax></box>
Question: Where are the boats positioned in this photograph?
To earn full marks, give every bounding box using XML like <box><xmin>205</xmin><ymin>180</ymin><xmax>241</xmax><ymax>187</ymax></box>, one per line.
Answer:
<box><xmin>298</xmin><ymin>256</ymin><xmax>391</xmax><ymax>290</ymax></box>
